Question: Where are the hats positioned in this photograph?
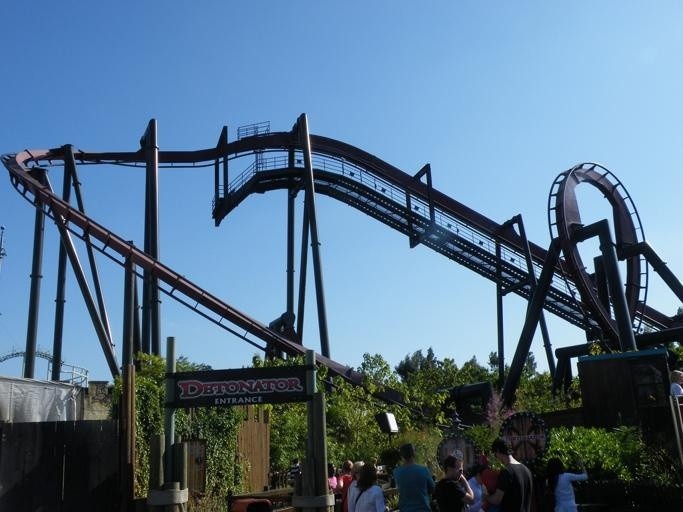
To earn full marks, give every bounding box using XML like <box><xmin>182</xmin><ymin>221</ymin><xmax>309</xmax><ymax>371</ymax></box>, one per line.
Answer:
<box><xmin>352</xmin><ymin>461</ymin><xmax>377</xmax><ymax>480</ymax></box>
<box><xmin>492</xmin><ymin>439</ymin><xmax>513</xmax><ymax>455</ymax></box>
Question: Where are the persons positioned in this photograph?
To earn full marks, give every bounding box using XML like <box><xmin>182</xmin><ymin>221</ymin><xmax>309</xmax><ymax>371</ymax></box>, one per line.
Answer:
<box><xmin>669</xmin><ymin>370</ymin><xmax>682</xmax><ymax>396</ymax></box>
<box><xmin>545</xmin><ymin>456</ymin><xmax>589</xmax><ymax>512</ymax></box>
<box><xmin>286</xmin><ymin>458</ymin><xmax>302</xmax><ymax>487</ymax></box>
<box><xmin>327</xmin><ymin>436</ymin><xmax>534</xmax><ymax>512</ymax></box>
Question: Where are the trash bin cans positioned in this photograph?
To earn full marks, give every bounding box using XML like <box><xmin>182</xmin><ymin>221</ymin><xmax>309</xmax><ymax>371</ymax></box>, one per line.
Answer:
<box><xmin>232</xmin><ymin>498</ymin><xmax>273</xmax><ymax>512</ymax></box>
